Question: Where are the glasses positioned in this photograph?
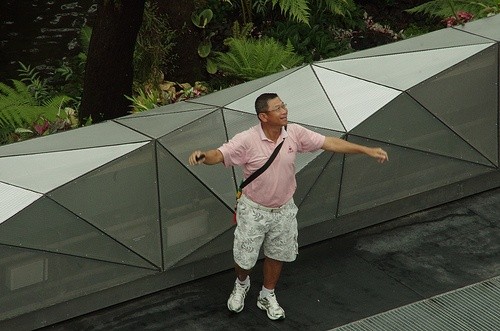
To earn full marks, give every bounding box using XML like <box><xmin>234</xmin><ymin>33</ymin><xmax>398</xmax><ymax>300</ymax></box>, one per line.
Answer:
<box><xmin>260</xmin><ymin>104</ymin><xmax>287</xmax><ymax>114</ymax></box>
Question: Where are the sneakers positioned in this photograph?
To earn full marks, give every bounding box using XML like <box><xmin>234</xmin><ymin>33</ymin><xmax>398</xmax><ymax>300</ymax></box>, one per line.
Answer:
<box><xmin>227</xmin><ymin>276</ymin><xmax>250</xmax><ymax>313</ymax></box>
<box><xmin>257</xmin><ymin>291</ymin><xmax>286</xmax><ymax>321</ymax></box>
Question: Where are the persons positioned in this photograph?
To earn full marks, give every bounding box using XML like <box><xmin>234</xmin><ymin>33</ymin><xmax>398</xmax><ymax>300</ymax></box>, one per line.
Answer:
<box><xmin>189</xmin><ymin>93</ymin><xmax>390</xmax><ymax>320</ymax></box>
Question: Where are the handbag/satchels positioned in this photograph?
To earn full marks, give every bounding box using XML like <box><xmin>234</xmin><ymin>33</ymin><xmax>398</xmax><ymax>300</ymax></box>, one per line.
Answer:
<box><xmin>233</xmin><ymin>179</ymin><xmax>244</xmax><ymax>225</ymax></box>
